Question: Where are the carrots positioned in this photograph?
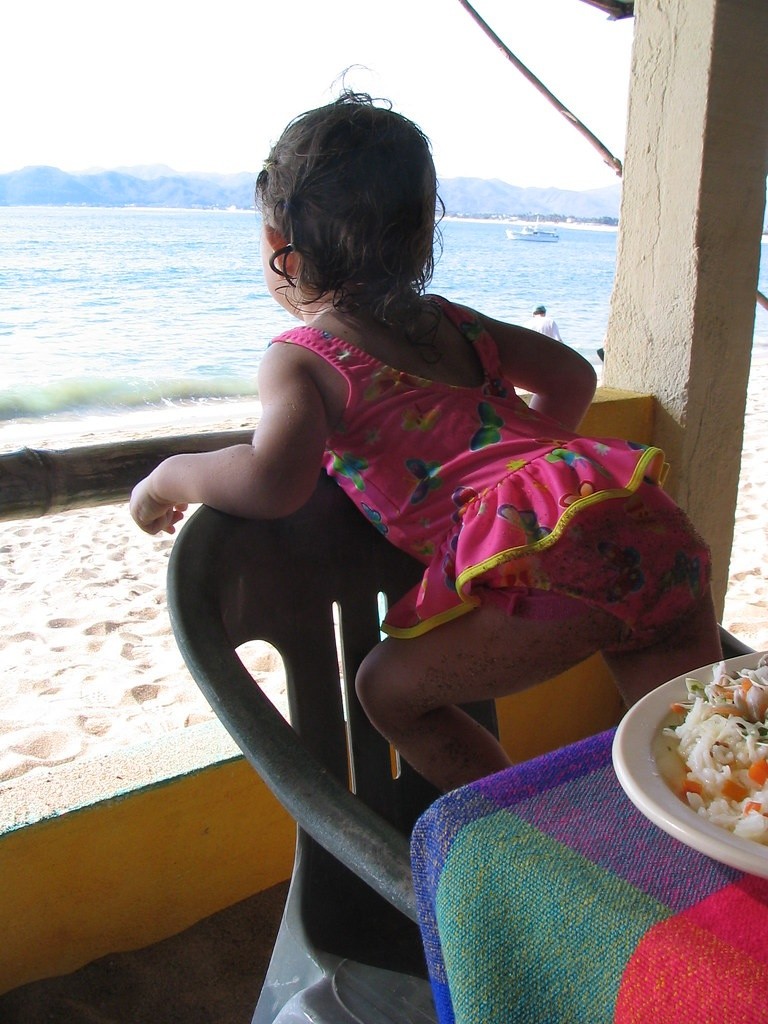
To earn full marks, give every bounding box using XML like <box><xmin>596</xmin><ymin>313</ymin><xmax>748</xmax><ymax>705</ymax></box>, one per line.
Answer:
<box><xmin>667</xmin><ymin>677</ymin><xmax>768</xmax><ymax>818</ymax></box>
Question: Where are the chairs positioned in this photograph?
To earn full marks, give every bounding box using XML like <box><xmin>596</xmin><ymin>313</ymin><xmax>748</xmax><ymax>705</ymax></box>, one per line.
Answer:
<box><xmin>168</xmin><ymin>466</ymin><xmax>757</xmax><ymax>1023</ymax></box>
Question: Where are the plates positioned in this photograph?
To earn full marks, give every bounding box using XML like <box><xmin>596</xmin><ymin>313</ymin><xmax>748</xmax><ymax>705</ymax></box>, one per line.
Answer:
<box><xmin>609</xmin><ymin>651</ymin><xmax>768</xmax><ymax>882</ymax></box>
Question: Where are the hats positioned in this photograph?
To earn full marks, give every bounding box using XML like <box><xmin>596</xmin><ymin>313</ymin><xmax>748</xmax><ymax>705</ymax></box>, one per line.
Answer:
<box><xmin>534</xmin><ymin>306</ymin><xmax>546</xmax><ymax>315</ymax></box>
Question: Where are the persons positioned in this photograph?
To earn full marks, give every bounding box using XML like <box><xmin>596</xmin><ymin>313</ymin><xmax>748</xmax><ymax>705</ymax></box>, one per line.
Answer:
<box><xmin>130</xmin><ymin>88</ymin><xmax>725</xmax><ymax>790</ymax></box>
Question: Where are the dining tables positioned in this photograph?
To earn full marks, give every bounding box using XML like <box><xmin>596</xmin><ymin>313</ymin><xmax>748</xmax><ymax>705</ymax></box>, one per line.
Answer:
<box><xmin>407</xmin><ymin>725</ymin><xmax>768</xmax><ymax>1024</ymax></box>
